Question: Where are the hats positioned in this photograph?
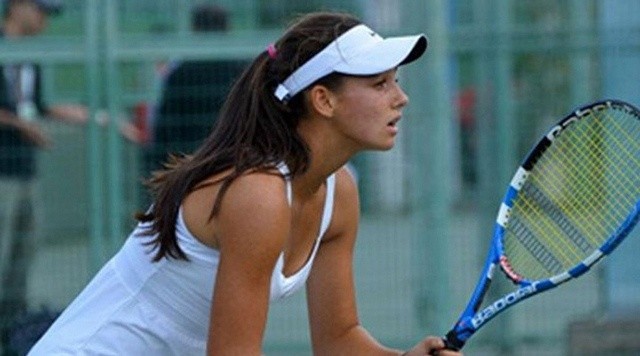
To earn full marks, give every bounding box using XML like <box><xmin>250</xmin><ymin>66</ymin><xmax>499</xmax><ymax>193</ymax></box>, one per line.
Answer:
<box><xmin>35</xmin><ymin>0</ymin><xmax>63</xmax><ymax>15</ymax></box>
<box><xmin>274</xmin><ymin>24</ymin><xmax>428</xmax><ymax>105</ymax></box>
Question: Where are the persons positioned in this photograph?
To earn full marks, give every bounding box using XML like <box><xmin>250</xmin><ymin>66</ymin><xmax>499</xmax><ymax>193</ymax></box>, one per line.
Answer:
<box><xmin>146</xmin><ymin>4</ymin><xmax>252</xmax><ymax>206</ymax></box>
<box><xmin>0</xmin><ymin>0</ymin><xmax>89</xmax><ymax>336</ymax></box>
<box><xmin>27</xmin><ymin>12</ymin><xmax>463</xmax><ymax>356</ymax></box>
<box><xmin>115</xmin><ymin>51</ymin><xmax>179</xmax><ymax>145</ymax></box>
